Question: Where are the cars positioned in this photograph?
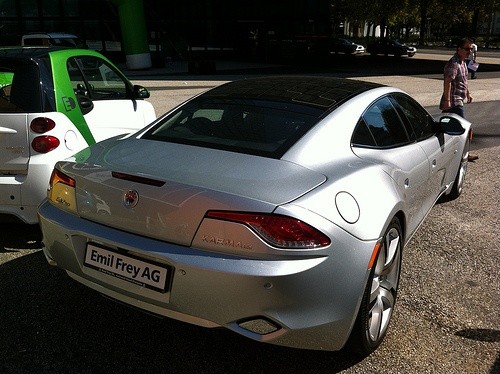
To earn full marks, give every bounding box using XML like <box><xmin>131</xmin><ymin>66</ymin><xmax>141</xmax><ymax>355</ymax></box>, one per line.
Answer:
<box><xmin>366</xmin><ymin>36</ymin><xmax>416</xmax><ymax>57</ymax></box>
<box><xmin>36</xmin><ymin>73</ymin><xmax>473</xmax><ymax>355</ymax></box>
<box><xmin>331</xmin><ymin>38</ymin><xmax>367</xmax><ymax>55</ymax></box>
<box><xmin>0</xmin><ymin>46</ymin><xmax>157</xmax><ymax>240</ymax></box>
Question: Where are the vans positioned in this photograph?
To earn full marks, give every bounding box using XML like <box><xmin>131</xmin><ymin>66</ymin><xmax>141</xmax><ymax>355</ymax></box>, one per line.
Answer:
<box><xmin>21</xmin><ymin>33</ymin><xmax>98</xmax><ymax>71</ymax></box>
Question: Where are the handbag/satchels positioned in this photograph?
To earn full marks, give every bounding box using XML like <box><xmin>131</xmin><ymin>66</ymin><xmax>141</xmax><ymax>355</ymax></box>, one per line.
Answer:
<box><xmin>468</xmin><ymin>60</ymin><xmax>479</xmax><ymax>72</ymax></box>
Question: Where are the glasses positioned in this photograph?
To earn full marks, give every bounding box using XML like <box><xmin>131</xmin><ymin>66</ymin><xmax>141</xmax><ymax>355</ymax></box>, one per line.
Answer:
<box><xmin>460</xmin><ymin>46</ymin><xmax>473</xmax><ymax>51</ymax></box>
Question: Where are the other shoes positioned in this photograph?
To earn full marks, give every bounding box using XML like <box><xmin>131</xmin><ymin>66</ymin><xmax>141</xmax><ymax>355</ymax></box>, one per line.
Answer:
<box><xmin>469</xmin><ymin>153</ymin><xmax>478</xmax><ymax>161</ymax></box>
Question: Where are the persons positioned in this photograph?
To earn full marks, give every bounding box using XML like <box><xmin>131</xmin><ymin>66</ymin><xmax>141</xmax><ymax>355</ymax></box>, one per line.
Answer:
<box><xmin>439</xmin><ymin>37</ymin><xmax>479</xmax><ymax>160</ymax></box>
<box><xmin>465</xmin><ymin>39</ymin><xmax>477</xmax><ymax>79</ymax></box>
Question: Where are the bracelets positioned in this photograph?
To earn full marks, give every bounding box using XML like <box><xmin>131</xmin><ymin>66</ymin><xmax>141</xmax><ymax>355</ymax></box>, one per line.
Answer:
<box><xmin>445</xmin><ymin>100</ymin><xmax>450</xmax><ymax>102</ymax></box>
<box><xmin>467</xmin><ymin>93</ymin><xmax>470</xmax><ymax>95</ymax></box>
